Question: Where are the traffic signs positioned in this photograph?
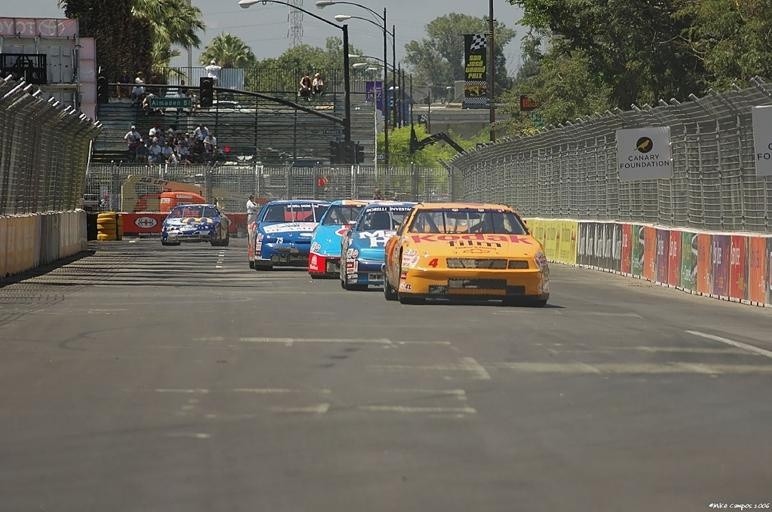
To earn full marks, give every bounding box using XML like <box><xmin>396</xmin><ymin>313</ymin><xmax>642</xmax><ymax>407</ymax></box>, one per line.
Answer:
<box><xmin>149</xmin><ymin>98</ymin><xmax>192</xmax><ymax>107</ymax></box>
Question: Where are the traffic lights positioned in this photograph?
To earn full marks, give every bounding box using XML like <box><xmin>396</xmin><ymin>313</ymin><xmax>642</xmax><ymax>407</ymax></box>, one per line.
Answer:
<box><xmin>199</xmin><ymin>76</ymin><xmax>214</xmax><ymax>107</ymax></box>
<box><xmin>339</xmin><ymin>117</ymin><xmax>350</xmax><ymax>143</ymax></box>
<box><xmin>329</xmin><ymin>139</ymin><xmax>341</xmax><ymax>165</ymax></box>
<box><xmin>343</xmin><ymin>143</ymin><xmax>354</xmax><ymax>165</ymax></box>
<box><xmin>95</xmin><ymin>72</ymin><xmax>109</xmax><ymax>104</ymax></box>
<box><xmin>353</xmin><ymin>143</ymin><xmax>365</xmax><ymax>164</ymax></box>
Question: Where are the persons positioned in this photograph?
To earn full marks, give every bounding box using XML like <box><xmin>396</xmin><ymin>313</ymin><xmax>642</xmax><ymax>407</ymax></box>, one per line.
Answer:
<box><xmin>116</xmin><ymin>69</ymin><xmax>198</xmax><ymax>117</ymax></box>
<box><xmin>245</xmin><ymin>193</ymin><xmax>262</xmax><ymax>225</ymax></box>
<box><xmin>297</xmin><ymin>74</ymin><xmax>312</xmax><ymax>102</ymax></box>
<box><xmin>312</xmin><ymin>71</ymin><xmax>324</xmax><ymax>96</ymax></box>
<box><xmin>124</xmin><ymin>122</ymin><xmax>217</xmax><ymax>166</ymax></box>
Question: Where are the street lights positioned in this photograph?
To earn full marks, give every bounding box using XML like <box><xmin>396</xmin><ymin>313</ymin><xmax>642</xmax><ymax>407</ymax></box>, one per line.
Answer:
<box><xmin>315</xmin><ymin>0</ymin><xmax>417</xmax><ymax>168</ymax></box>
<box><xmin>237</xmin><ymin>0</ymin><xmax>351</xmax><ymax>145</ymax></box>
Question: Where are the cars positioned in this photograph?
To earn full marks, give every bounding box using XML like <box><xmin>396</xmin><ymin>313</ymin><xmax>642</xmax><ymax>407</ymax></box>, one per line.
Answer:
<box><xmin>159</xmin><ymin>203</ymin><xmax>232</xmax><ymax>248</ymax></box>
<box><xmin>248</xmin><ymin>198</ymin><xmax>553</xmax><ymax>308</ymax></box>
